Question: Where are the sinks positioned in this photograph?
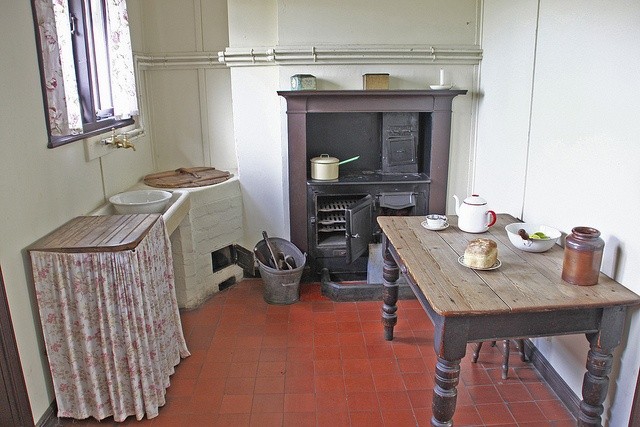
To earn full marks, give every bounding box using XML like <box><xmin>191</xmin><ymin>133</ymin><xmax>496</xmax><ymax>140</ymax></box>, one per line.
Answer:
<box><xmin>94</xmin><ymin>189</ymin><xmax>182</xmax><ymax>219</ymax></box>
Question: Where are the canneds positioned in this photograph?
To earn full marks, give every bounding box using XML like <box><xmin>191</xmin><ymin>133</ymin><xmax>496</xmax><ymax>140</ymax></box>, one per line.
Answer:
<box><xmin>562</xmin><ymin>227</ymin><xmax>605</xmax><ymax>286</ymax></box>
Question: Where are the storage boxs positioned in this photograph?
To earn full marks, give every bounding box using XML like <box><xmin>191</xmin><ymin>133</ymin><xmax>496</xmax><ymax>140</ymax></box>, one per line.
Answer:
<box><xmin>363</xmin><ymin>74</ymin><xmax>389</xmax><ymax>90</ymax></box>
<box><xmin>291</xmin><ymin>74</ymin><xmax>316</xmax><ymax>93</ymax></box>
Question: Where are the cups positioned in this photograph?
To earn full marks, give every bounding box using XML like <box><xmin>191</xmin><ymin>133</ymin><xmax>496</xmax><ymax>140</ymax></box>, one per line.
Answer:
<box><xmin>427</xmin><ymin>214</ymin><xmax>447</xmax><ymax>227</ymax></box>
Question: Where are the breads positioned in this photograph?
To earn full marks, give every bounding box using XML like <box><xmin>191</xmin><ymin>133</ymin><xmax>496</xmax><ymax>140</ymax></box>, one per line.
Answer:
<box><xmin>464</xmin><ymin>238</ymin><xmax>497</xmax><ymax>269</ymax></box>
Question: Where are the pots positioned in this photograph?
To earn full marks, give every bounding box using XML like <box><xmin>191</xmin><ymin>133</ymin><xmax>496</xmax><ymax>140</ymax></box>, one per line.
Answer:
<box><xmin>310</xmin><ymin>153</ymin><xmax>360</xmax><ymax>181</ymax></box>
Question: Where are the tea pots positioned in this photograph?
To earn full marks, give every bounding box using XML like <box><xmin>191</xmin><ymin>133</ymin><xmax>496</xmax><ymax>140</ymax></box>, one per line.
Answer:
<box><xmin>454</xmin><ymin>194</ymin><xmax>495</xmax><ymax>233</ymax></box>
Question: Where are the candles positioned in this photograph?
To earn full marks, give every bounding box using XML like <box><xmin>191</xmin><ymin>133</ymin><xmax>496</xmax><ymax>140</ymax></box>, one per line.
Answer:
<box><xmin>439</xmin><ymin>69</ymin><xmax>444</xmax><ymax>86</ymax></box>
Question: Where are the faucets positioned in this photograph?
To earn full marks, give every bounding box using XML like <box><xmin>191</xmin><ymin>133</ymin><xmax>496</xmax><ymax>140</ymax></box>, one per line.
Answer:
<box><xmin>118</xmin><ymin>133</ymin><xmax>134</xmax><ymax>151</ymax></box>
<box><xmin>106</xmin><ymin>127</ymin><xmax>118</xmax><ymax>149</ymax></box>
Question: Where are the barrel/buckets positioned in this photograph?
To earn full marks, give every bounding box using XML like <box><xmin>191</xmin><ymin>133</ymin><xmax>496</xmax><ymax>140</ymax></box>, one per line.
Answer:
<box><xmin>254</xmin><ymin>238</ymin><xmax>306</xmax><ymax>307</ymax></box>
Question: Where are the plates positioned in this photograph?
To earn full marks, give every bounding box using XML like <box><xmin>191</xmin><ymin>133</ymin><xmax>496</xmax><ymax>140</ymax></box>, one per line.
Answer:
<box><xmin>457</xmin><ymin>256</ymin><xmax>502</xmax><ymax>270</ymax></box>
<box><xmin>421</xmin><ymin>221</ymin><xmax>450</xmax><ymax>231</ymax></box>
<box><xmin>430</xmin><ymin>84</ymin><xmax>452</xmax><ymax>89</ymax></box>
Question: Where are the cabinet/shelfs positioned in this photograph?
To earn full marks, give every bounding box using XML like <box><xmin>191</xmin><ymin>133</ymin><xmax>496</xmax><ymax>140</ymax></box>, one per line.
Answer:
<box><xmin>278</xmin><ymin>88</ymin><xmax>469</xmax><ymax>282</ymax></box>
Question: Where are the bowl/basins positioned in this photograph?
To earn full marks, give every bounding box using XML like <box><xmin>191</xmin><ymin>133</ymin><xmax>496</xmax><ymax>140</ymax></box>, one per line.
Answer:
<box><xmin>109</xmin><ymin>190</ymin><xmax>173</xmax><ymax>214</ymax></box>
<box><xmin>506</xmin><ymin>222</ymin><xmax>561</xmax><ymax>253</ymax></box>
